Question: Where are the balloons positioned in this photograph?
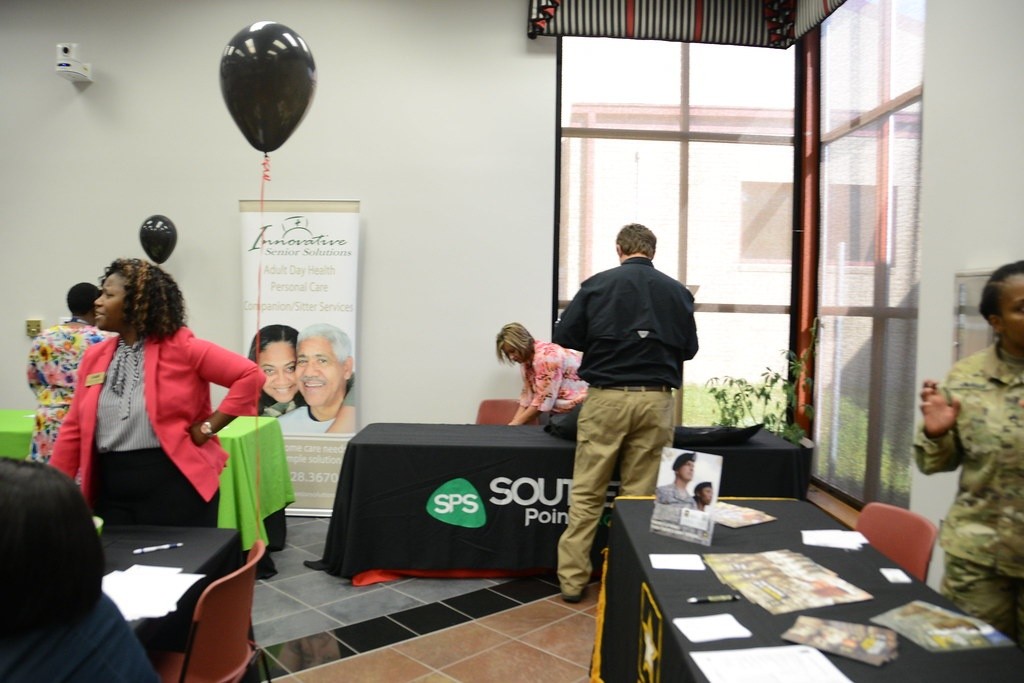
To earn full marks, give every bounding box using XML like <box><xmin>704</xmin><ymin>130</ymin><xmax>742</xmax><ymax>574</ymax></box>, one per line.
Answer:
<box><xmin>220</xmin><ymin>21</ymin><xmax>317</xmax><ymax>153</ymax></box>
<box><xmin>140</xmin><ymin>214</ymin><xmax>177</xmax><ymax>264</ymax></box>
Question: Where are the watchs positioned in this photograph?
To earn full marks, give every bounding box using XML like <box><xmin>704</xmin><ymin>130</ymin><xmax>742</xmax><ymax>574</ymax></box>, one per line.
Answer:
<box><xmin>201</xmin><ymin>422</ymin><xmax>214</xmax><ymax>438</ymax></box>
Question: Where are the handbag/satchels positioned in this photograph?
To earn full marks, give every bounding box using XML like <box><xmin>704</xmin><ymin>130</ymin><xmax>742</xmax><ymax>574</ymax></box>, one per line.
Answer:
<box><xmin>544</xmin><ymin>400</ymin><xmax>582</xmax><ymax>441</ymax></box>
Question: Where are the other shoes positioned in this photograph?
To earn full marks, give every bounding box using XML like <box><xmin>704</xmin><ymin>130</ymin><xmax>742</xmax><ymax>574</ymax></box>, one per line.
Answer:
<box><xmin>563</xmin><ymin>587</ymin><xmax>587</xmax><ymax>603</ymax></box>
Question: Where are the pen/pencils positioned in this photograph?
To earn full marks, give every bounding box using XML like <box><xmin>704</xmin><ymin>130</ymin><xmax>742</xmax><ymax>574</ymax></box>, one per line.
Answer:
<box><xmin>687</xmin><ymin>594</ymin><xmax>741</xmax><ymax>603</ymax></box>
<box><xmin>132</xmin><ymin>543</ymin><xmax>184</xmax><ymax>554</ymax></box>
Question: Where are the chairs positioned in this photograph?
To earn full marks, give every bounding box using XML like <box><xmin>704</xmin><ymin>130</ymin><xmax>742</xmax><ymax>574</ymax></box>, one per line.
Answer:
<box><xmin>853</xmin><ymin>500</ymin><xmax>938</xmax><ymax>584</ymax></box>
<box><xmin>478</xmin><ymin>397</ymin><xmax>541</xmax><ymax>424</ymax></box>
<box><xmin>146</xmin><ymin>534</ymin><xmax>272</xmax><ymax>683</ymax></box>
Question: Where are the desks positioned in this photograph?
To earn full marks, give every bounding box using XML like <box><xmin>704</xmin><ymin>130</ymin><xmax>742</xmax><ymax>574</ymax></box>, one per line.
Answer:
<box><xmin>103</xmin><ymin>523</ymin><xmax>261</xmax><ymax>683</ymax></box>
<box><xmin>0</xmin><ymin>407</ymin><xmax>297</xmax><ymax>579</ymax></box>
<box><xmin>303</xmin><ymin>423</ymin><xmax>811</xmax><ymax>586</ymax></box>
<box><xmin>588</xmin><ymin>495</ymin><xmax>1024</xmax><ymax>683</ymax></box>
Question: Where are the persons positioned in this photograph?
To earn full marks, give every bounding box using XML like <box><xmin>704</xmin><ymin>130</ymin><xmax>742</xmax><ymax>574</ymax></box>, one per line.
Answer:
<box><xmin>496</xmin><ymin>322</ymin><xmax>589</xmax><ymax>425</ymax></box>
<box><xmin>28</xmin><ymin>282</ymin><xmax>113</xmax><ymax>460</ymax></box>
<box><xmin>250</xmin><ymin>325</ymin><xmax>355</xmax><ymax>433</ymax></box>
<box><xmin>46</xmin><ymin>256</ymin><xmax>267</xmax><ymax>526</ymax></box>
<box><xmin>553</xmin><ymin>223</ymin><xmax>699</xmax><ymax>603</ymax></box>
<box><xmin>693</xmin><ymin>482</ymin><xmax>713</xmax><ymax>511</ymax></box>
<box><xmin>0</xmin><ymin>456</ymin><xmax>158</xmax><ymax>683</ymax></box>
<box><xmin>277</xmin><ymin>322</ymin><xmax>353</xmax><ymax>434</ymax></box>
<box><xmin>657</xmin><ymin>450</ymin><xmax>696</xmax><ymax>510</ymax></box>
<box><xmin>912</xmin><ymin>258</ymin><xmax>1024</xmax><ymax>619</ymax></box>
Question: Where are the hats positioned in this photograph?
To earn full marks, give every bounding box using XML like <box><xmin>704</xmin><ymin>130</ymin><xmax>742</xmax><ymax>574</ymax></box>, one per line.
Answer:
<box><xmin>673</xmin><ymin>453</ymin><xmax>697</xmax><ymax>470</ymax></box>
<box><xmin>694</xmin><ymin>482</ymin><xmax>713</xmax><ymax>495</ymax></box>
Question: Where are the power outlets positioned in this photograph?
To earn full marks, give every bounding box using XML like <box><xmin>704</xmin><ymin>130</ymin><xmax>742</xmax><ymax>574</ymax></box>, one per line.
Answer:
<box><xmin>26</xmin><ymin>320</ymin><xmax>41</xmax><ymax>337</ymax></box>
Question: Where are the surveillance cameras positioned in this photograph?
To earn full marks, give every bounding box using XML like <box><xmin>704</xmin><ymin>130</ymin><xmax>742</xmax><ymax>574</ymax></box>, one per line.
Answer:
<box><xmin>56</xmin><ymin>43</ymin><xmax>82</xmax><ymax>64</ymax></box>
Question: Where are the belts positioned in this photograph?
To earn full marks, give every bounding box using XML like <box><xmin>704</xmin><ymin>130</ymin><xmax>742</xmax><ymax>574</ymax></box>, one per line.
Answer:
<box><xmin>587</xmin><ymin>381</ymin><xmax>672</xmax><ymax>393</ymax></box>
